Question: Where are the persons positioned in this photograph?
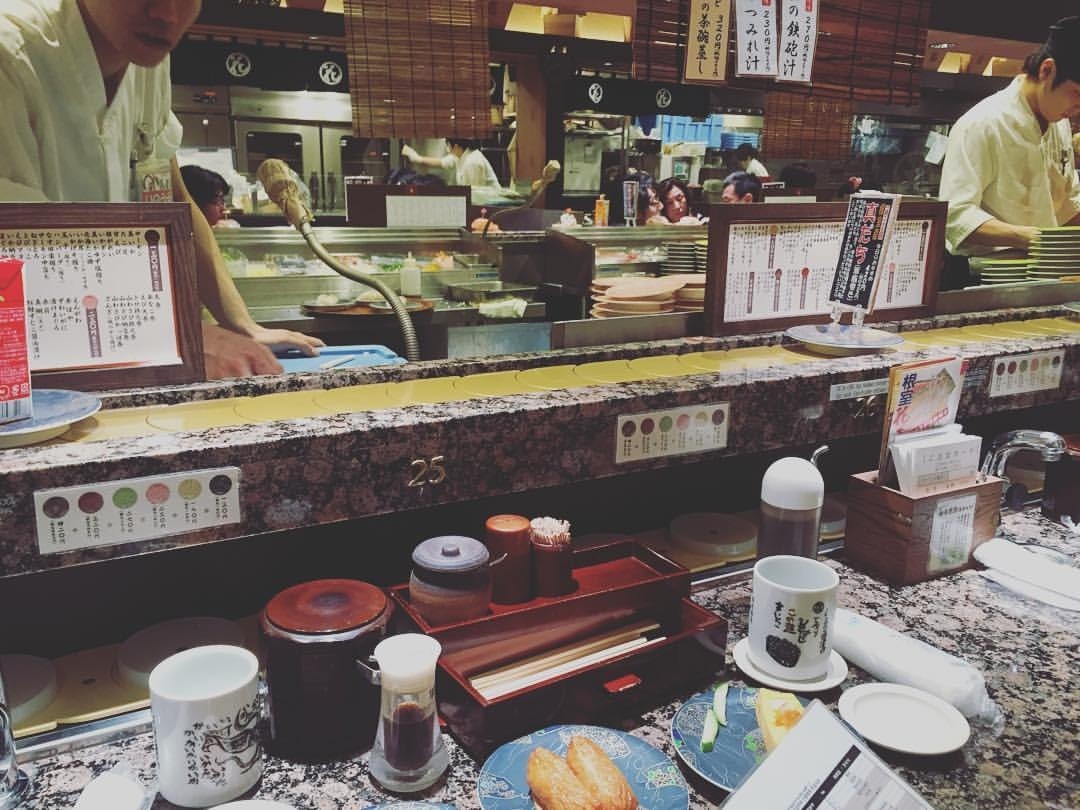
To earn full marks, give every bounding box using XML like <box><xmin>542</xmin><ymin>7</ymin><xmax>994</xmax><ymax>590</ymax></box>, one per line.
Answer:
<box><xmin>604</xmin><ymin>165</ymin><xmax>709</xmax><ymax>225</ymax></box>
<box><xmin>387</xmin><ymin>136</ymin><xmax>502</xmax><ymax>188</ymax></box>
<box><xmin>938</xmin><ymin>16</ymin><xmax>1080</xmax><ymax>283</ymax></box>
<box><xmin>0</xmin><ymin>0</ymin><xmax>327</xmax><ymax>381</ymax></box>
<box><xmin>178</xmin><ymin>164</ymin><xmax>231</xmax><ymax>227</ymax></box>
<box><xmin>722</xmin><ymin>171</ymin><xmax>763</xmax><ymax>204</ymax></box>
<box><xmin>735</xmin><ymin>142</ymin><xmax>767</xmax><ymax>177</ymax></box>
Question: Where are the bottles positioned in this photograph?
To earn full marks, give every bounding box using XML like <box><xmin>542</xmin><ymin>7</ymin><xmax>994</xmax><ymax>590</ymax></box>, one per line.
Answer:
<box><xmin>400</xmin><ymin>251</ymin><xmax>421</xmax><ymax>298</ymax></box>
<box><xmin>486</xmin><ymin>515</ymin><xmax>531</xmax><ymax>604</ymax></box>
<box><xmin>409</xmin><ymin>534</ymin><xmax>492</xmax><ymax>627</ymax></box>
<box><xmin>368</xmin><ymin>633</ymin><xmax>449</xmax><ymax>792</ymax></box>
<box><xmin>755</xmin><ymin>456</ymin><xmax>824</xmax><ymax>560</ymax></box>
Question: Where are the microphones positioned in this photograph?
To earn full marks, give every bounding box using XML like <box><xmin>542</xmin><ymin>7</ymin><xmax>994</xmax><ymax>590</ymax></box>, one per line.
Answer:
<box><xmin>257</xmin><ymin>156</ymin><xmax>421</xmax><ymax>361</ymax></box>
<box><xmin>482</xmin><ymin>159</ymin><xmax>561</xmax><ymax>241</ymax></box>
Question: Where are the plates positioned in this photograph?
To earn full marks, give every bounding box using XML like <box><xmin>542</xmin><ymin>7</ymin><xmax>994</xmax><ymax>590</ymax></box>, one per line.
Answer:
<box><xmin>476</xmin><ymin>725</ymin><xmax>689</xmax><ymax>810</ymax></box>
<box><xmin>663</xmin><ymin>241</ymin><xmax>696</xmax><ymax>274</ymax></box>
<box><xmin>368</xmin><ymin>302</ymin><xmax>423</xmax><ymax>310</ymax></box>
<box><xmin>672</xmin><ymin>273</ymin><xmax>706</xmax><ymax>311</ymax></box>
<box><xmin>1025</xmin><ymin>226</ymin><xmax>1080</xmax><ymax>279</ymax></box>
<box><xmin>733</xmin><ymin>636</ymin><xmax>848</xmax><ymax>692</ymax></box>
<box><xmin>0</xmin><ymin>389</ymin><xmax>101</xmax><ymax>448</ymax></box>
<box><xmin>979</xmin><ymin>260</ymin><xmax>1032</xmax><ymax>282</ymax></box>
<box><xmin>694</xmin><ymin>240</ymin><xmax>708</xmax><ymax>273</ymax></box>
<box><xmin>589</xmin><ymin>277</ymin><xmax>682</xmax><ymax>318</ymax></box>
<box><xmin>302</xmin><ymin>300</ymin><xmax>355</xmax><ymax>312</ymax></box>
<box><xmin>837</xmin><ymin>683</ymin><xmax>971</xmax><ymax>755</ymax></box>
<box><xmin>670</xmin><ymin>687</ymin><xmax>811</xmax><ymax>792</ymax></box>
<box><xmin>354</xmin><ymin>298</ymin><xmax>400</xmax><ymax>304</ymax></box>
<box><xmin>784</xmin><ymin>324</ymin><xmax>903</xmax><ymax>357</ymax></box>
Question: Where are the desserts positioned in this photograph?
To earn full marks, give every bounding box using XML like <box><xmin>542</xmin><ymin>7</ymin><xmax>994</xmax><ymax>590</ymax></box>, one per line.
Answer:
<box><xmin>757</xmin><ymin>691</ymin><xmax>804</xmax><ymax>751</ymax></box>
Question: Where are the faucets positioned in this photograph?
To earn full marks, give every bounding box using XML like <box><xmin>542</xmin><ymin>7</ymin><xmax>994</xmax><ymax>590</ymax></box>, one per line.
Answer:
<box><xmin>980</xmin><ymin>427</ymin><xmax>1066</xmax><ymax>502</ymax></box>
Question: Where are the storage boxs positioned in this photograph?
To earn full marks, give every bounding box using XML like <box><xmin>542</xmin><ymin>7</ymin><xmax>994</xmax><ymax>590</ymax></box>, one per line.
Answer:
<box><xmin>847</xmin><ymin>469</ymin><xmax>1007</xmax><ymax>589</ymax></box>
<box><xmin>437</xmin><ymin>597</ymin><xmax>728</xmax><ymax>766</ymax></box>
<box><xmin>389</xmin><ymin>539</ymin><xmax>692</xmax><ymax>638</ymax></box>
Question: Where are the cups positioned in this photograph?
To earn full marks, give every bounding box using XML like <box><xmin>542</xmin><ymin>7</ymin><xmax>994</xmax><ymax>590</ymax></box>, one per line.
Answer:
<box><xmin>148</xmin><ymin>645</ymin><xmax>262</xmax><ymax>808</ymax></box>
<box><xmin>746</xmin><ymin>555</ymin><xmax>839</xmax><ymax>680</ymax></box>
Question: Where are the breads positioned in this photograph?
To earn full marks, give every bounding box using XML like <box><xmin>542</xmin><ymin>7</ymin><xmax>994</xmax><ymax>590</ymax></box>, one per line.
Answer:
<box><xmin>526</xmin><ymin>735</ymin><xmax>638</xmax><ymax>810</ymax></box>
